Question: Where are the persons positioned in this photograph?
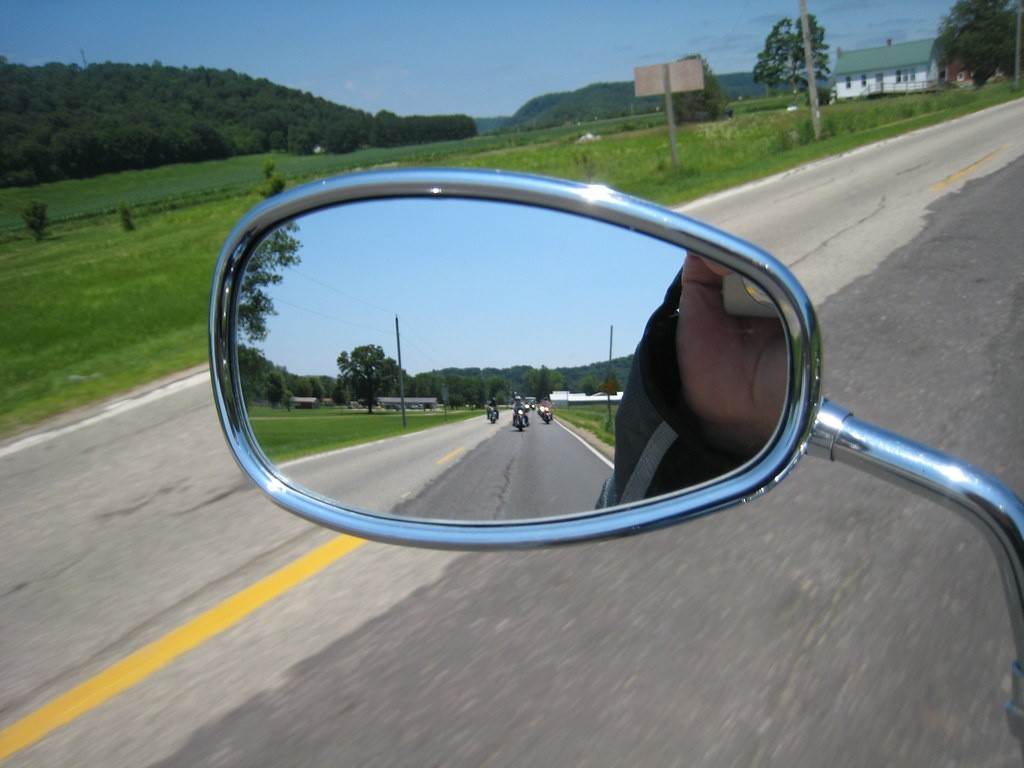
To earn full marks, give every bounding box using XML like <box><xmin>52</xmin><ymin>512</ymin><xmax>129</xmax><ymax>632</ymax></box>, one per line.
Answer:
<box><xmin>487</xmin><ymin>395</ymin><xmax>554</xmax><ymax>426</ymax></box>
<box><xmin>594</xmin><ymin>250</ymin><xmax>790</xmax><ymax>512</ymax></box>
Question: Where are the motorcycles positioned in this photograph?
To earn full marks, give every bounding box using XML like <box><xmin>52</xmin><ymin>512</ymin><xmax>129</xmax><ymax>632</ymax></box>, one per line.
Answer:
<box><xmin>538</xmin><ymin>406</ymin><xmax>552</xmax><ymax>423</ymax></box>
<box><xmin>524</xmin><ymin>404</ymin><xmax>536</xmax><ymax>410</ymax></box>
<box><xmin>488</xmin><ymin>406</ymin><xmax>498</xmax><ymax>423</ymax></box>
<box><xmin>513</xmin><ymin>407</ymin><xmax>528</xmax><ymax>432</ymax></box>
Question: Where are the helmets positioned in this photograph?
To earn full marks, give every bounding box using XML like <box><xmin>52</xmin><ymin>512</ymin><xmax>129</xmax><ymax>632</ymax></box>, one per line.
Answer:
<box><xmin>515</xmin><ymin>396</ymin><xmax>521</xmax><ymax>401</ymax></box>
<box><xmin>491</xmin><ymin>398</ymin><xmax>496</xmax><ymax>401</ymax></box>
